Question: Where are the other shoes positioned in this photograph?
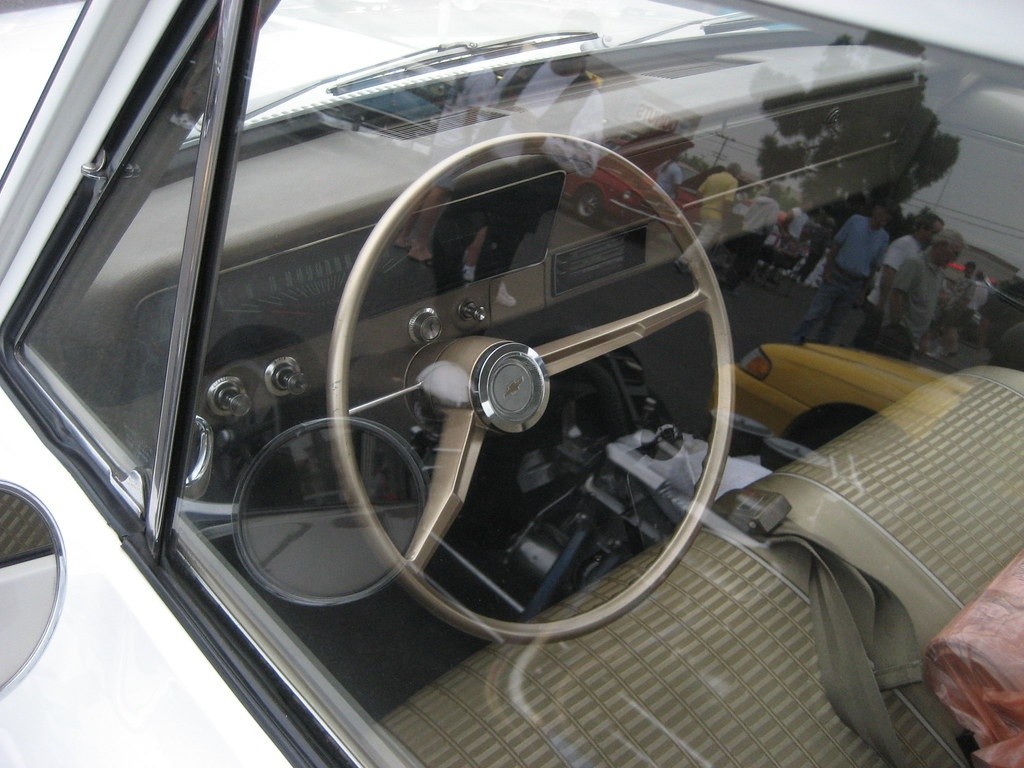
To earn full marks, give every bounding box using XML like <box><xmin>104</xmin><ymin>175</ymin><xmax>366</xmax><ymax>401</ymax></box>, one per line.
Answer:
<box><xmin>674</xmin><ymin>260</ymin><xmax>684</xmax><ymax>273</ymax></box>
<box><xmin>463</xmin><ymin>265</ymin><xmax>475</xmax><ymax>281</ymax></box>
<box><xmin>496</xmin><ymin>284</ymin><xmax>518</xmax><ymax>306</ymax></box>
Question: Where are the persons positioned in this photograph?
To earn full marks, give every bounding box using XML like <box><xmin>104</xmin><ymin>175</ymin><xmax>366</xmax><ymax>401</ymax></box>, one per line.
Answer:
<box><xmin>648</xmin><ymin>147</ymin><xmax>989</xmax><ymax>365</ymax></box>
<box><xmin>395</xmin><ymin>7</ymin><xmax>604</xmax><ymax>329</ymax></box>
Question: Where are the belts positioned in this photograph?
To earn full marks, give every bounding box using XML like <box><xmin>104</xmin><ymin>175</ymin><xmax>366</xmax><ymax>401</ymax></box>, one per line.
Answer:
<box><xmin>834</xmin><ymin>262</ymin><xmax>864</xmax><ymax>283</ymax></box>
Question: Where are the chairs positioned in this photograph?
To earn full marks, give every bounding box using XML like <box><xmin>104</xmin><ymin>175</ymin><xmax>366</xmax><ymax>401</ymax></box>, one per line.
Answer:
<box><xmin>379</xmin><ymin>526</ymin><xmax>968</xmax><ymax>768</ymax></box>
<box><xmin>739</xmin><ymin>364</ymin><xmax>1024</xmax><ymax>738</ymax></box>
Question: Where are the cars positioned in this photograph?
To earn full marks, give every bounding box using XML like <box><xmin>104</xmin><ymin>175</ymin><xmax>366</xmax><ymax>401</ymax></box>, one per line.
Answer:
<box><xmin>559</xmin><ymin>157</ymin><xmax>696</xmax><ymax>237</ymax></box>
<box><xmin>711</xmin><ymin>333</ymin><xmax>969</xmax><ymax>475</ymax></box>
<box><xmin>981</xmin><ymin>272</ymin><xmax>1024</xmax><ymax>320</ymax></box>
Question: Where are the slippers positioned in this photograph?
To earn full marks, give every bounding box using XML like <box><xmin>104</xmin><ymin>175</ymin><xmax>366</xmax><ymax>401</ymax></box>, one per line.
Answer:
<box><xmin>394</xmin><ymin>241</ymin><xmax>410</xmax><ymax>249</ymax></box>
<box><xmin>408</xmin><ymin>254</ymin><xmax>433</xmax><ymax>266</ymax></box>
